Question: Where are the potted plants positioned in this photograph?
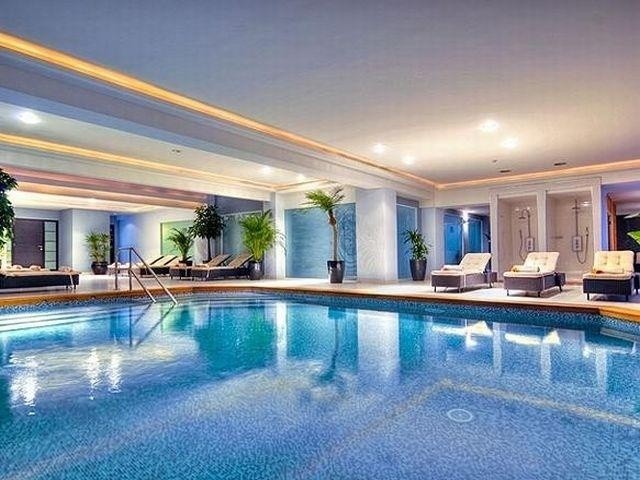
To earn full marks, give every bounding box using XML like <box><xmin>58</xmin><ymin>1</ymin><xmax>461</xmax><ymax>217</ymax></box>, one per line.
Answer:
<box><xmin>189</xmin><ymin>202</ymin><xmax>227</xmax><ymax>265</ymax></box>
<box><xmin>298</xmin><ymin>182</ymin><xmax>349</xmax><ymax>285</ymax></box>
<box><xmin>236</xmin><ymin>206</ymin><xmax>288</xmax><ymax>279</ymax></box>
<box><xmin>83</xmin><ymin>227</ymin><xmax>115</xmax><ymax>276</ymax></box>
<box><xmin>163</xmin><ymin>225</ymin><xmax>195</xmax><ymax>278</ymax></box>
<box><xmin>399</xmin><ymin>225</ymin><xmax>434</xmax><ymax>283</ymax></box>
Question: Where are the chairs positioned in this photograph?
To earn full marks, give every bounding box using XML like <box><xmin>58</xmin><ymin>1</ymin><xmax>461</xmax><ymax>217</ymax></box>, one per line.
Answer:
<box><xmin>104</xmin><ymin>252</ymin><xmax>256</xmax><ymax>283</ymax></box>
<box><xmin>429</xmin><ymin>251</ymin><xmax>498</xmax><ymax>293</ymax></box>
<box><xmin>502</xmin><ymin>249</ymin><xmax>566</xmax><ymax>298</ymax></box>
<box><xmin>581</xmin><ymin>249</ymin><xmax>637</xmax><ymax>301</ymax></box>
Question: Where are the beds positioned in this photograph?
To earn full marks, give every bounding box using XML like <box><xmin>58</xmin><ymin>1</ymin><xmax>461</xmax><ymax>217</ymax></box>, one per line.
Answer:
<box><xmin>0</xmin><ymin>263</ymin><xmax>83</xmax><ymax>293</ymax></box>
